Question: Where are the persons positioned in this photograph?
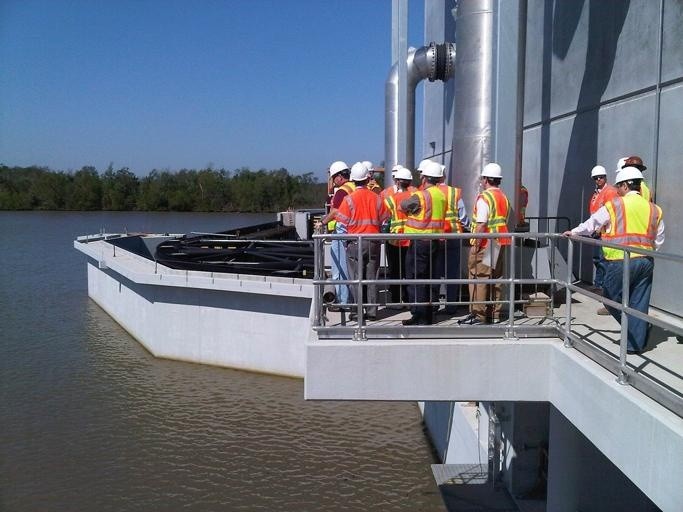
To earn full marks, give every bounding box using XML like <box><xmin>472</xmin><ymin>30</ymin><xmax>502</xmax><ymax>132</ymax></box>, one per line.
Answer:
<box><xmin>517</xmin><ymin>186</ymin><xmax>527</xmax><ymax>226</ymax></box>
<box><xmin>560</xmin><ymin>167</ymin><xmax>665</xmax><ymax>354</ymax></box>
<box><xmin>597</xmin><ymin>156</ymin><xmax>630</xmax><ymax>302</ymax></box>
<box><xmin>314</xmin><ymin>156</ymin><xmax>510</xmax><ymax>325</ymax></box>
<box><xmin>588</xmin><ymin>165</ymin><xmax>617</xmax><ymax>294</ymax></box>
<box><xmin>597</xmin><ymin>156</ymin><xmax>652</xmax><ymax>315</ymax></box>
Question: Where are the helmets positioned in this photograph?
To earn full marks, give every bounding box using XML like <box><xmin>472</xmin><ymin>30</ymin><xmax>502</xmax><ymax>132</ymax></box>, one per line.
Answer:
<box><xmin>350</xmin><ymin>162</ymin><xmax>368</xmax><ymax>182</ymax></box>
<box><xmin>392</xmin><ymin>165</ymin><xmax>404</xmax><ymax>176</ymax></box>
<box><xmin>328</xmin><ymin>161</ymin><xmax>350</xmax><ymax>179</ymax></box>
<box><xmin>481</xmin><ymin>162</ymin><xmax>504</xmax><ymax>179</ymax></box>
<box><xmin>612</xmin><ymin>166</ymin><xmax>645</xmax><ymax>188</ymax></box>
<box><xmin>419</xmin><ymin>162</ymin><xmax>447</xmax><ymax>178</ymax></box>
<box><xmin>615</xmin><ymin>156</ymin><xmax>629</xmax><ymax>174</ymax></box>
<box><xmin>416</xmin><ymin>159</ymin><xmax>433</xmax><ymax>171</ymax></box>
<box><xmin>590</xmin><ymin>165</ymin><xmax>607</xmax><ymax>179</ymax></box>
<box><xmin>394</xmin><ymin>168</ymin><xmax>414</xmax><ymax>181</ymax></box>
<box><xmin>361</xmin><ymin>161</ymin><xmax>375</xmax><ymax>172</ymax></box>
<box><xmin>621</xmin><ymin>155</ymin><xmax>647</xmax><ymax>171</ymax></box>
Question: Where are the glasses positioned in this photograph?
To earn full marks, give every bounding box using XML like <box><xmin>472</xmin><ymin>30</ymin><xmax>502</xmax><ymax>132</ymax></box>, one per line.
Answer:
<box><xmin>594</xmin><ymin>176</ymin><xmax>604</xmax><ymax>180</ymax></box>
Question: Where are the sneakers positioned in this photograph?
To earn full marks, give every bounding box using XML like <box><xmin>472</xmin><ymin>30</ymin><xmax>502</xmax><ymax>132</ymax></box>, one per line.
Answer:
<box><xmin>402</xmin><ymin>315</ymin><xmax>433</xmax><ymax>326</ymax></box>
<box><xmin>486</xmin><ymin>312</ymin><xmax>504</xmax><ymax>323</ymax></box>
<box><xmin>457</xmin><ymin>313</ymin><xmax>487</xmax><ymax>326</ymax></box>
